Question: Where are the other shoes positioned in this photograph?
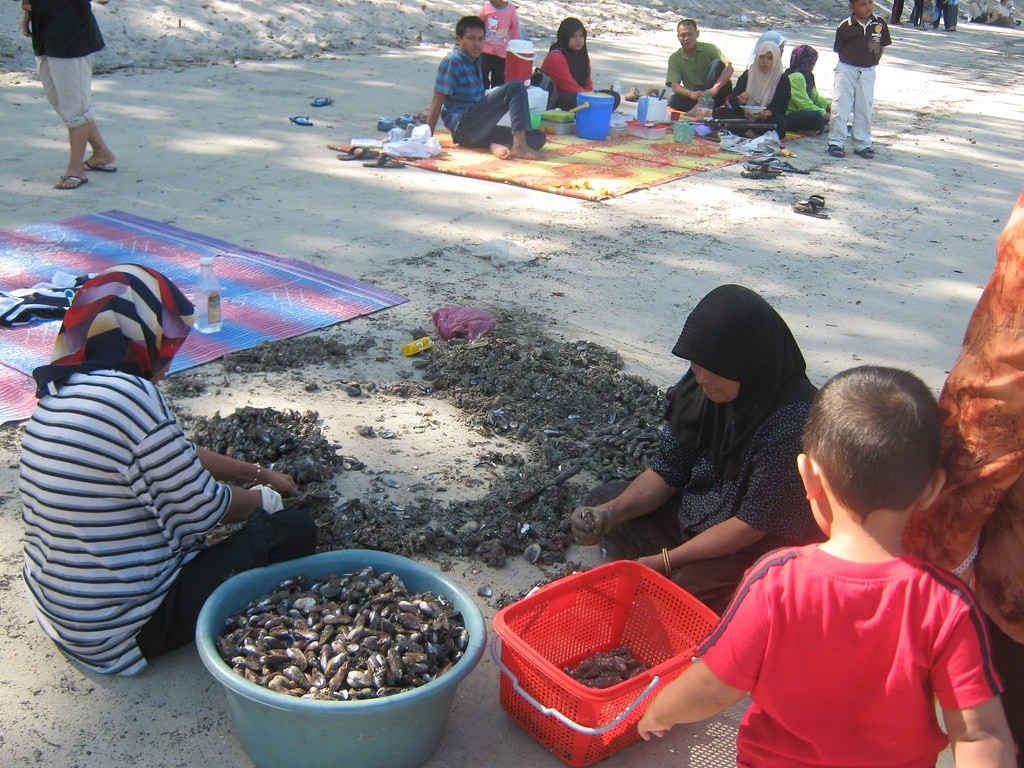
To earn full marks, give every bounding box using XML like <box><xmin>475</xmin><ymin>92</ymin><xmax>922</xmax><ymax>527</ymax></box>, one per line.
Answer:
<box><xmin>648</xmin><ymin>88</ymin><xmax>659</xmax><ymax>98</ymax></box>
<box><xmin>625</xmin><ymin>87</ymin><xmax>639</xmax><ymax>101</ymax></box>
<box><xmin>914</xmin><ymin>26</ymin><xmax>927</xmax><ymax>31</ymax></box>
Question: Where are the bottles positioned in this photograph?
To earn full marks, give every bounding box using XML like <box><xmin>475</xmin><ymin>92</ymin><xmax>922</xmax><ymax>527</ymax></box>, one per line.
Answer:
<box><xmin>696</xmin><ymin>93</ymin><xmax>714</xmax><ymax>120</ymax></box>
<box><xmin>193</xmin><ymin>258</ymin><xmax>223</xmax><ymax>333</ymax></box>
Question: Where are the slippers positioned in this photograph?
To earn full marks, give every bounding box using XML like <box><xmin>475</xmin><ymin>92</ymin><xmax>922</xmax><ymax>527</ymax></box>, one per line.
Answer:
<box><xmin>854</xmin><ymin>147</ymin><xmax>875</xmax><ymax>159</ymax></box>
<box><xmin>337</xmin><ymin>145</ymin><xmax>379</xmax><ymax>160</ymax></box>
<box><xmin>749</xmin><ymin>158</ymin><xmax>810</xmax><ymax>174</ymax></box>
<box><xmin>794</xmin><ymin>195</ymin><xmax>828</xmax><ymax>219</ymax></box>
<box><xmin>54</xmin><ymin>176</ymin><xmax>88</xmax><ymax>189</ymax></box>
<box><xmin>378</xmin><ymin>112</ymin><xmax>428</xmax><ymax>132</ymax></box>
<box><xmin>289</xmin><ymin>115</ymin><xmax>313</xmax><ymax>126</ymax></box>
<box><xmin>83</xmin><ymin>160</ymin><xmax>117</xmax><ymax>172</ymax></box>
<box><xmin>828</xmin><ymin>144</ymin><xmax>845</xmax><ymax>158</ymax></box>
<box><xmin>311</xmin><ymin>97</ymin><xmax>332</xmax><ymax>106</ymax></box>
<box><xmin>740</xmin><ymin>164</ymin><xmax>782</xmax><ymax>179</ymax></box>
<box><xmin>362</xmin><ymin>155</ymin><xmax>406</xmax><ymax>168</ymax></box>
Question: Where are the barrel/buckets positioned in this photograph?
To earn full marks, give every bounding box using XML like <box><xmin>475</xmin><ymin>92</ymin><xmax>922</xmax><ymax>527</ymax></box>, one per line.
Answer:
<box><xmin>569</xmin><ymin>91</ymin><xmax>614</xmax><ymax>141</ymax></box>
<box><xmin>507</xmin><ymin>40</ymin><xmax>537</xmax><ymax>88</ymax></box>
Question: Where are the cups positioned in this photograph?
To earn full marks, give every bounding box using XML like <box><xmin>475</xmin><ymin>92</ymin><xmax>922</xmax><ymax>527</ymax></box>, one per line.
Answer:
<box><xmin>671</xmin><ymin>112</ymin><xmax>681</xmax><ymax>121</ymax></box>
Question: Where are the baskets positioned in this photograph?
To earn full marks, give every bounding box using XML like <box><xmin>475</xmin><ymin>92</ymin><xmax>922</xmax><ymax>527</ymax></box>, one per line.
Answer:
<box><xmin>490</xmin><ymin>560</ymin><xmax>722</xmax><ymax>768</ymax></box>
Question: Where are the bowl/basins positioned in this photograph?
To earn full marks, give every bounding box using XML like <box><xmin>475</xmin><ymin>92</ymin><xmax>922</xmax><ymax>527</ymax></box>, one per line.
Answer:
<box><xmin>196</xmin><ymin>550</ymin><xmax>488</xmax><ymax>767</ymax></box>
<box><xmin>608</xmin><ymin>121</ymin><xmax>628</xmax><ymax>136</ymax></box>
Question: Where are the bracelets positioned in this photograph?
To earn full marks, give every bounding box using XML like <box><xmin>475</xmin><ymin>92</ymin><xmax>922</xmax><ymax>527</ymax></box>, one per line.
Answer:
<box><xmin>244</xmin><ymin>462</ymin><xmax>261</xmax><ymax>488</ymax></box>
<box><xmin>662</xmin><ymin>548</ymin><xmax>672</xmax><ymax>577</ymax></box>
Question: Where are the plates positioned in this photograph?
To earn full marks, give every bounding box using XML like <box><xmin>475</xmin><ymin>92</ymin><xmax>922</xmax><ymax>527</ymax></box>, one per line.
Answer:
<box><xmin>740</xmin><ymin>105</ymin><xmax>767</xmax><ymax>114</ymax></box>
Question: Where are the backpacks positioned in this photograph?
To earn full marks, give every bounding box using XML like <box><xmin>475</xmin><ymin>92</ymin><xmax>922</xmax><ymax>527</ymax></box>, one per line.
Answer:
<box><xmin>531</xmin><ymin>67</ymin><xmax>558</xmax><ymax>108</ymax></box>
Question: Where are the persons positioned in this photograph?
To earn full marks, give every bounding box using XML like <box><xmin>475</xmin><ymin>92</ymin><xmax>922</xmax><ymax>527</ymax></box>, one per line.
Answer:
<box><xmin>890</xmin><ymin>0</ymin><xmax>959</xmax><ymax>32</ymax></box>
<box><xmin>18</xmin><ymin>0</ymin><xmax>118</xmax><ymax>190</ymax></box>
<box><xmin>569</xmin><ymin>284</ymin><xmax>829</xmax><ymax>619</ymax></box>
<box><xmin>425</xmin><ymin>0</ymin><xmax>621</xmax><ymax>161</ymax></box>
<box><xmin>900</xmin><ymin>185</ymin><xmax>1024</xmax><ymax>768</ymax></box>
<box><xmin>665</xmin><ymin>19</ymin><xmax>850</xmax><ymax>141</ymax></box>
<box><xmin>636</xmin><ymin>365</ymin><xmax>1018</xmax><ymax>768</ymax></box>
<box><xmin>827</xmin><ymin>0</ymin><xmax>893</xmax><ymax>159</ymax></box>
<box><xmin>967</xmin><ymin>0</ymin><xmax>1021</xmax><ymax>27</ymax></box>
<box><xmin>18</xmin><ymin>263</ymin><xmax>317</xmax><ymax>679</ymax></box>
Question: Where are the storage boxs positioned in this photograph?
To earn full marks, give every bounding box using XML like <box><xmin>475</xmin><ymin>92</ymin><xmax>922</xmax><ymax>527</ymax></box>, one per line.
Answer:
<box><xmin>625</xmin><ymin>120</ymin><xmax>668</xmax><ymax>140</ymax></box>
<box><xmin>541</xmin><ymin>119</ymin><xmax>576</xmax><ymax>135</ymax></box>
